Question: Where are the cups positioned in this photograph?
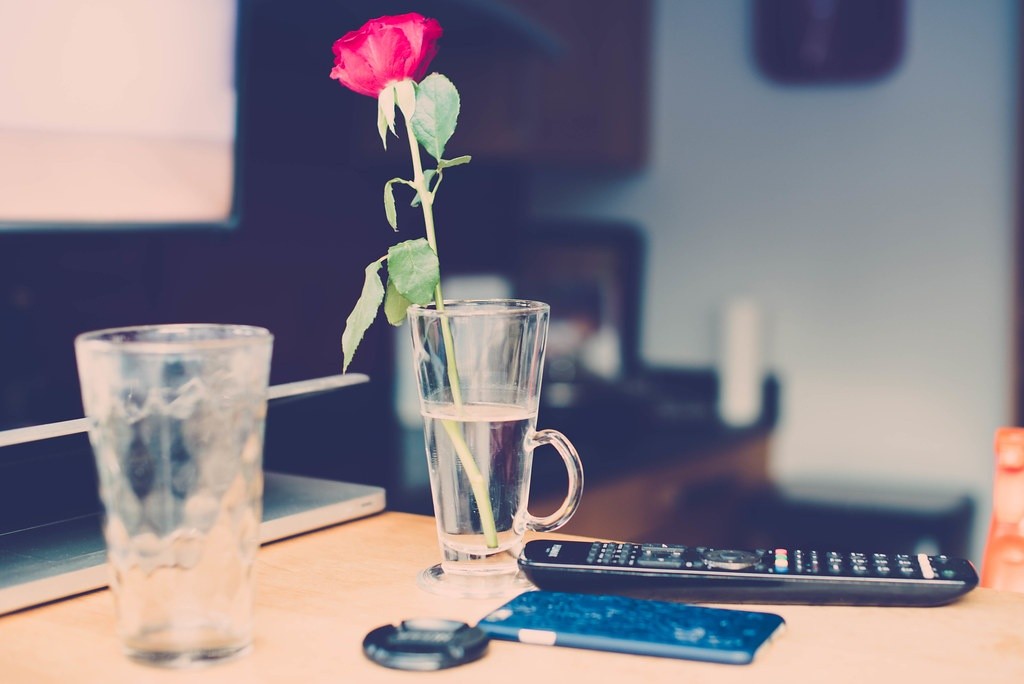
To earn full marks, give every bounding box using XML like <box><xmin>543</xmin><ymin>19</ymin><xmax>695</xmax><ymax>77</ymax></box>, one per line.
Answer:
<box><xmin>408</xmin><ymin>298</ymin><xmax>585</xmax><ymax>578</ymax></box>
<box><xmin>72</xmin><ymin>324</ymin><xmax>275</xmax><ymax>671</ymax></box>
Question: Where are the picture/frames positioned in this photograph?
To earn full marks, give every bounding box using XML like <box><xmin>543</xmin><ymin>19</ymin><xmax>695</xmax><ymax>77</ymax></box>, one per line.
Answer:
<box><xmin>524</xmin><ymin>220</ymin><xmax>646</xmax><ymax>375</ymax></box>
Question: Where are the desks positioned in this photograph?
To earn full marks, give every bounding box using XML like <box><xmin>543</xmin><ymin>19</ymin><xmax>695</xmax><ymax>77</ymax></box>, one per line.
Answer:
<box><xmin>0</xmin><ymin>511</ymin><xmax>1024</xmax><ymax>684</ymax></box>
<box><xmin>527</xmin><ymin>369</ymin><xmax>778</xmax><ymax>540</ymax></box>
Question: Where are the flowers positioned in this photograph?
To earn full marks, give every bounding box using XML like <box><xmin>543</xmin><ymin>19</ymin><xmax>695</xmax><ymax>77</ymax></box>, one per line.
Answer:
<box><xmin>329</xmin><ymin>12</ymin><xmax>498</xmax><ymax>548</ymax></box>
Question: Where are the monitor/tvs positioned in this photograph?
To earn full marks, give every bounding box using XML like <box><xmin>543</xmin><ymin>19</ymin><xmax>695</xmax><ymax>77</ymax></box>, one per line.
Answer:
<box><xmin>1</xmin><ymin>0</ymin><xmax>248</xmax><ymax>230</ymax></box>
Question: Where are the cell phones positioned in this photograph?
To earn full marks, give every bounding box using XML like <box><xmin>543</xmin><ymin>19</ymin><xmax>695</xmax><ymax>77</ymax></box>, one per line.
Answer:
<box><xmin>473</xmin><ymin>589</ymin><xmax>786</xmax><ymax>667</ymax></box>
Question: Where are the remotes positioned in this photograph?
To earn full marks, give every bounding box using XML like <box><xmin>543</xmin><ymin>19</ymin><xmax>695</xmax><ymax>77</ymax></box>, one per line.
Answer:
<box><xmin>517</xmin><ymin>537</ymin><xmax>981</xmax><ymax>605</ymax></box>
